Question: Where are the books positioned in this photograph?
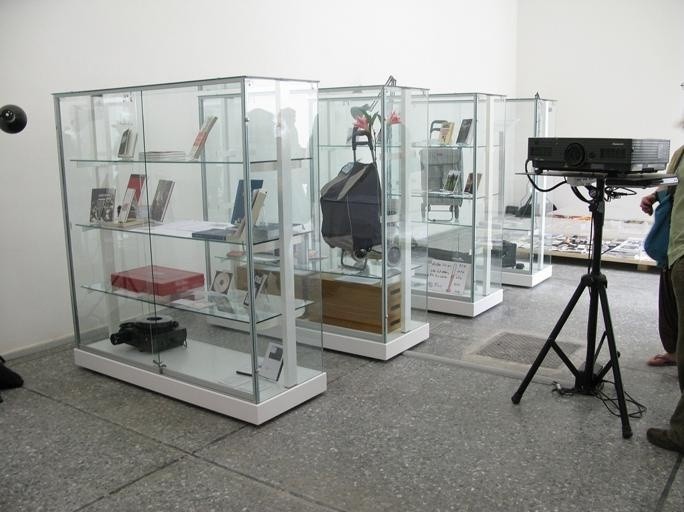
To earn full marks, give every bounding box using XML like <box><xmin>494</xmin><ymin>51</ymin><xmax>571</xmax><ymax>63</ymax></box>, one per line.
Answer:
<box><xmin>437</xmin><ymin>121</ymin><xmax>454</xmax><ymax>145</ymax></box>
<box><xmin>258</xmin><ymin>342</ymin><xmax>284</xmax><ymax>382</ymax></box>
<box><xmin>243</xmin><ymin>269</ymin><xmax>266</xmax><ymax>308</ymax></box>
<box><xmin>89</xmin><ymin>173</ymin><xmax>175</xmax><ymax>230</ymax></box>
<box><xmin>189</xmin><ymin>115</ymin><xmax>218</xmax><ymax>158</ymax></box>
<box><xmin>190</xmin><ymin>188</ymin><xmax>303</xmax><ymax>243</ymax></box>
<box><xmin>463</xmin><ymin>172</ymin><xmax>483</xmax><ymax>196</ymax></box>
<box><xmin>118</xmin><ymin>128</ymin><xmax>185</xmax><ymax>161</ymax></box>
<box><xmin>456</xmin><ymin>119</ymin><xmax>475</xmax><ymax>145</ymax></box>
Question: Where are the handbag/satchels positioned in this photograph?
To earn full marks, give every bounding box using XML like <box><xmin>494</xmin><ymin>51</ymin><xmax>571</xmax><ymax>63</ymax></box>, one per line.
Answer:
<box><xmin>644</xmin><ymin>193</ymin><xmax>672</xmax><ymax>261</ymax></box>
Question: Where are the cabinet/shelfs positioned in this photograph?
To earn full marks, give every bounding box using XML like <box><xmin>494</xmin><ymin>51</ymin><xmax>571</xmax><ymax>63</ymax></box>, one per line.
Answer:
<box><xmin>304</xmin><ymin>90</ymin><xmax>507</xmax><ymax>320</ymax></box>
<box><xmin>194</xmin><ymin>82</ymin><xmax>432</xmax><ymax>362</ymax></box>
<box><xmin>380</xmin><ymin>91</ymin><xmax>554</xmax><ymax>289</ymax></box>
<box><xmin>51</xmin><ymin>73</ymin><xmax>331</xmax><ymax>427</ymax></box>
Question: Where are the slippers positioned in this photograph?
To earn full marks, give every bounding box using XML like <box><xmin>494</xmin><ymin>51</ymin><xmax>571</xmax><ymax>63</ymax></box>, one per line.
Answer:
<box><xmin>646</xmin><ymin>354</ymin><xmax>680</xmax><ymax>367</ymax></box>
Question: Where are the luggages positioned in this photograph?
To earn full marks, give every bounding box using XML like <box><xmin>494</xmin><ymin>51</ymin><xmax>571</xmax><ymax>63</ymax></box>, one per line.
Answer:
<box><xmin>319</xmin><ymin>129</ymin><xmax>384</xmax><ymax>263</ymax></box>
<box><xmin>420</xmin><ymin>119</ymin><xmax>464</xmax><ymax>222</ymax></box>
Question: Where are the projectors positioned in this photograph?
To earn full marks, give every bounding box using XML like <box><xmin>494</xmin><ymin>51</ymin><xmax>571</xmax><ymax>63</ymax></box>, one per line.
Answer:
<box><xmin>528</xmin><ymin>139</ymin><xmax>671</xmax><ymax>178</ymax></box>
<box><xmin>109</xmin><ymin>314</ymin><xmax>187</xmax><ymax>353</ymax></box>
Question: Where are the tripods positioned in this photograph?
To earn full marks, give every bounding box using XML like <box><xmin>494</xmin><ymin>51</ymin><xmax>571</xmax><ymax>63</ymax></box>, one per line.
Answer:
<box><xmin>514</xmin><ymin>178</ymin><xmax>633</xmax><ymax>440</ymax></box>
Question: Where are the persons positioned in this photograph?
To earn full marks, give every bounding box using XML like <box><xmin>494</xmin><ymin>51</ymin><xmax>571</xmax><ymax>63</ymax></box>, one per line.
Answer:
<box><xmin>645</xmin><ymin>145</ymin><xmax>684</xmax><ymax>453</ymax></box>
<box><xmin>639</xmin><ymin>187</ymin><xmax>679</xmax><ymax>367</ymax></box>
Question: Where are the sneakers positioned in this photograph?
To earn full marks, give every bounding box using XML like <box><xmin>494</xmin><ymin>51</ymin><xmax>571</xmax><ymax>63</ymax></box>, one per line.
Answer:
<box><xmin>646</xmin><ymin>427</ymin><xmax>684</xmax><ymax>453</ymax></box>
<box><xmin>1</xmin><ymin>363</ymin><xmax>24</xmax><ymax>389</ymax></box>
